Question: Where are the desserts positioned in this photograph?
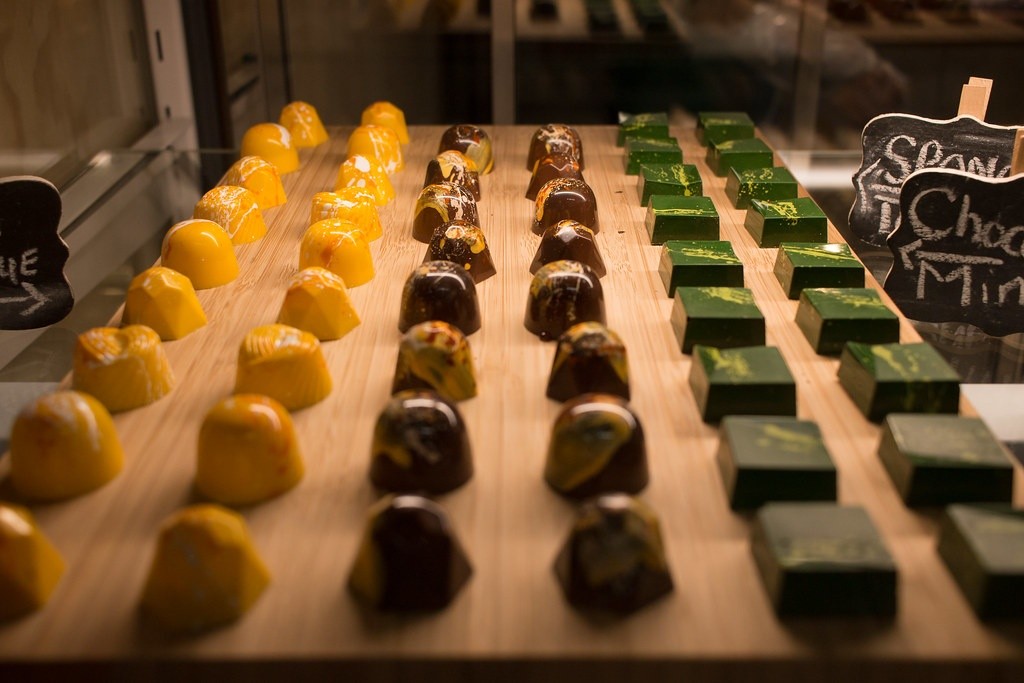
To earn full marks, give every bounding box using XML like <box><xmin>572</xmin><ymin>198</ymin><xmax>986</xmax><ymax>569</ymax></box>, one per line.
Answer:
<box><xmin>0</xmin><ymin>100</ymin><xmax>1024</xmax><ymax>629</ymax></box>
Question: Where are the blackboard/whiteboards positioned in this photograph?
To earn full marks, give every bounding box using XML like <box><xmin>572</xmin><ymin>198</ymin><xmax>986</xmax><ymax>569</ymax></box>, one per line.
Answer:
<box><xmin>0</xmin><ymin>172</ymin><xmax>77</xmax><ymax>332</ymax></box>
<box><xmin>844</xmin><ymin>112</ymin><xmax>1023</xmax><ymax>338</ymax></box>
